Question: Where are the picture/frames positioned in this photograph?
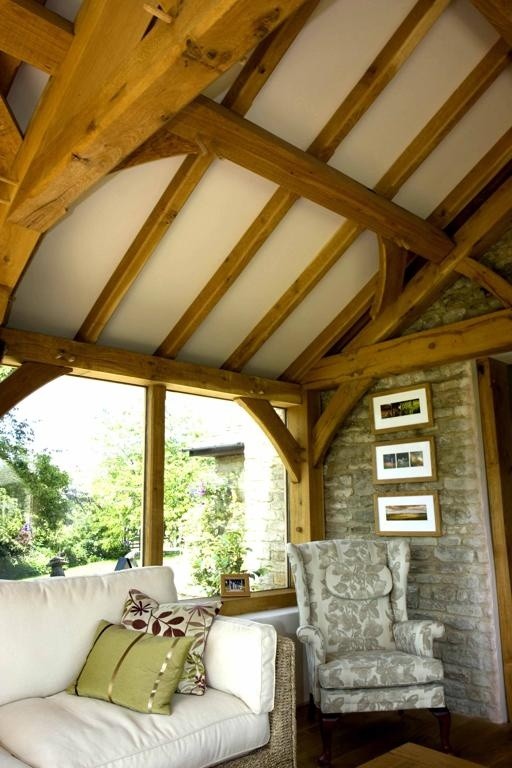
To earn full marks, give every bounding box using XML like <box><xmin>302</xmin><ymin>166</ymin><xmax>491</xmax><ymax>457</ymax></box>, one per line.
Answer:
<box><xmin>369</xmin><ymin>381</ymin><xmax>443</xmax><ymax>537</ymax></box>
<box><xmin>221</xmin><ymin>574</ymin><xmax>251</xmax><ymax>597</ymax></box>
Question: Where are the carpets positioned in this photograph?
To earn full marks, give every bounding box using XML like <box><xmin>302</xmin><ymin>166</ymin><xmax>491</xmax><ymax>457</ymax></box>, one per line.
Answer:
<box><xmin>356</xmin><ymin>741</ymin><xmax>486</xmax><ymax>768</ymax></box>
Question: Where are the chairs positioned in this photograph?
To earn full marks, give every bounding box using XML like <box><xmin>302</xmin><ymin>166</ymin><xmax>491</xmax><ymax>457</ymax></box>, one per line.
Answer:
<box><xmin>286</xmin><ymin>538</ymin><xmax>452</xmax><ymax>768</ymax></box>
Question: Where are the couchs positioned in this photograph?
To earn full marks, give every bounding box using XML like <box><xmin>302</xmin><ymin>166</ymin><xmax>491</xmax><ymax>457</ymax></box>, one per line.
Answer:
<box><xmin>0</xmin><ymin>566</ymin><xmax>296</xmax><ymax>768</ymax></box>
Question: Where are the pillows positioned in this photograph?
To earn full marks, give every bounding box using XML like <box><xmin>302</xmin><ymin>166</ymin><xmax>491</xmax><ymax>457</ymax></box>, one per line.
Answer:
<box><xmin>66</xmin><ymin>589</ymin><xmax>224</xmax><ymax>715</ymax></box>
